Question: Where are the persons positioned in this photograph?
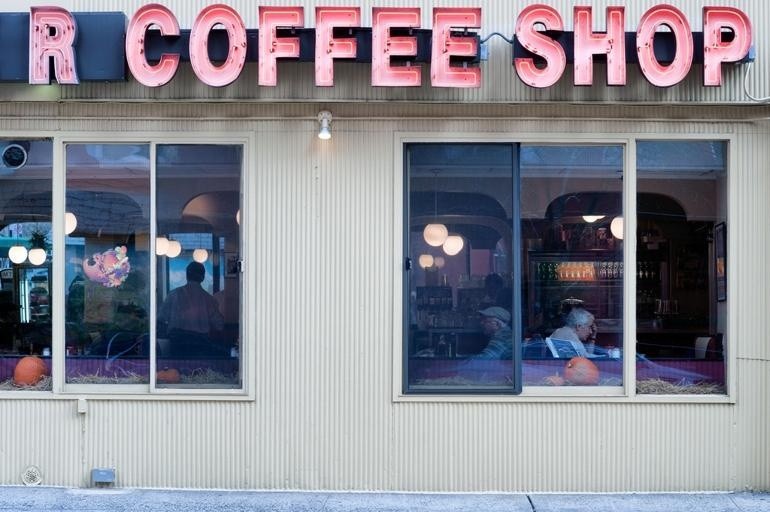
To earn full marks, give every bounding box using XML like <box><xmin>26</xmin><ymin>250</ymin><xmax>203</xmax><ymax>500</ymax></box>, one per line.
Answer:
<box><xmin>461</xmin><ymin>304</ymin><xmax>513</xmax><ymax>386</ymax></box>
<box><xmin>157</xmin><ymin>261</ymin><xmax>226</xmax><ymax>359</ymax></box>
<box><xmin>539</xmin><ymin>308</ymin><xmax>621</xmax><ymax>363</ymax></box>
<box><xmin>478</xmin><ymin>274</ymin><xmax>513</xmax><ymax>309</ymax></box>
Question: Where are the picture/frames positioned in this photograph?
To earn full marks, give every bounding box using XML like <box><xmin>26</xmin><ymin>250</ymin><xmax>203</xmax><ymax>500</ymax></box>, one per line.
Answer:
<box><xmin>714</xmin><ymin>222</ymin><xmax>727</xmax><ymax>303</ymax></box>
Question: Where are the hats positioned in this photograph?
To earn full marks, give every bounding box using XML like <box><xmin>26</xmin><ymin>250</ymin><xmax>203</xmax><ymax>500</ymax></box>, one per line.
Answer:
<box><xmin>477</xmin><ymin>306</ymin><xmax>512</xmax><ymax>323</ymax></box>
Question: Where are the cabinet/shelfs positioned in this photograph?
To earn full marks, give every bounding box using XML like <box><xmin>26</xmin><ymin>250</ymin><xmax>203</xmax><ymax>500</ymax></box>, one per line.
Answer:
<box><xmin>527</xmin><ymin>250</ymin><xmax>665</xmax><ymax>332</ymax></box>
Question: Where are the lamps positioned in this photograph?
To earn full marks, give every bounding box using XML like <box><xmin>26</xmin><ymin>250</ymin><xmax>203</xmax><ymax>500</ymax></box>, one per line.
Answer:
<box><xmin>317</xmin><ymin>110</ymin><xmax>334</xmax><ymax>142</ymax></box>
<box><xmin>7</xmin><ymin>219</ymin><xmax>47</xmax><ymax>268</ymax></box>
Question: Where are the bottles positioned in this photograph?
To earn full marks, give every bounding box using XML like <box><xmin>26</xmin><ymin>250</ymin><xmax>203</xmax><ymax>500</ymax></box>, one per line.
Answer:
<box><xmin>612</xmin><ymin>345</ymin><xmax>621</xmax><ymax>359</ymax></box>
<box><xmin>539</xmin><ymin>262</ymin><xmax>624</xmax><ymax>281</ymax></box>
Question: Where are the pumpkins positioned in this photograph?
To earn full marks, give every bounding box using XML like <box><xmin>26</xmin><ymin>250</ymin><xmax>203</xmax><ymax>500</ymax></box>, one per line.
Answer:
<box><xmin>549</xmin><ymin>371</ymin><xmax>565</xmax><ymax>386</ymax></box>
<box><xmin>14</xmin><ymin>356</ymin><xmax>47</xmax><ymax>386</ymax></box>
<box><xmin>565</xmin><ymin>357</ymin><xmax>600</xmax><ymax>386</ymax></box>
<box><xmin>157</xmin><ymin>367</ymin><xmax>179</xmax><ymax>384</ymax></box>
